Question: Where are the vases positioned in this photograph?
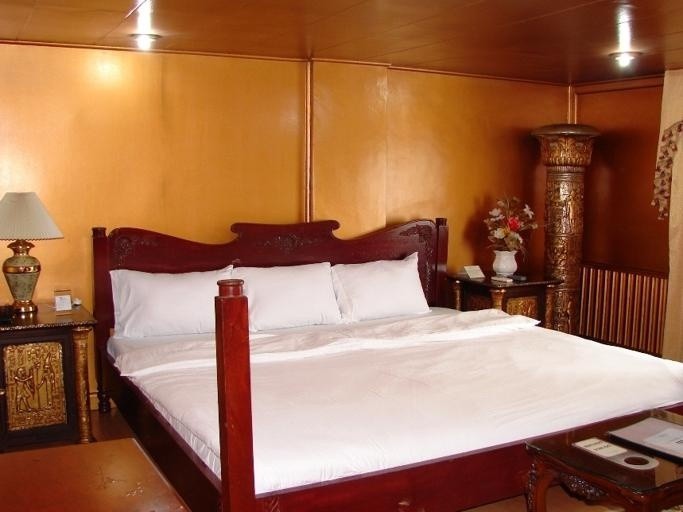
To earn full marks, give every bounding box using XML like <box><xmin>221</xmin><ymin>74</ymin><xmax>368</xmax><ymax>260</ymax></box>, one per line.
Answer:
<box><xmin>491</xmin><ymin>250</ymin><xmax>518</xmax><ymax>277</ymax></box>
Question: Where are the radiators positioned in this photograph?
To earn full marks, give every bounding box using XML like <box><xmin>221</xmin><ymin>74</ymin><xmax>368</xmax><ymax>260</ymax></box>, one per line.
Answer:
<box><xmin>576</xmin><ymin>265</ymin><xmax>670</xmax><ymax>357</ymax></box>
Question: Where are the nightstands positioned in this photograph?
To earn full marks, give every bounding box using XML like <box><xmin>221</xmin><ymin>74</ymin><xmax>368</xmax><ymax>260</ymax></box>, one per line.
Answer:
<box><xmin>0</xmin><ymin>299</ymin><xmax>99</xmax><ymax>444</ymax></box>
<box><xmin>449</xmin><ymin>270</ymin><xmax>564</xmax><ymax>328</ymax></box>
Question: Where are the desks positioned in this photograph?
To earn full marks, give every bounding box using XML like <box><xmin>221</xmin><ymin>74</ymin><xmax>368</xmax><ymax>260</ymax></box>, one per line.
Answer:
<box><xmin>521</xmin><ymin>408</ymin><xmax>683</xmax><ymax>512</ymax></box>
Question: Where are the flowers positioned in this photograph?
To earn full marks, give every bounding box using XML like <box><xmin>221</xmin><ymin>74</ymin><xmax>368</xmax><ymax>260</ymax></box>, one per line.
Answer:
<box><xmin>481</xmin><ymin>191</ymin><xmax>538</xmax><ymax>263</ymax></box>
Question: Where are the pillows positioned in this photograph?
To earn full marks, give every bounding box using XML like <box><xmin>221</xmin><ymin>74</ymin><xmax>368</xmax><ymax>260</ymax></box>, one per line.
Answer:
<box><xmin>231</xmin><ymin>260</ymin><xmax>342</xmax><ymax>333</ymax></box>
<box><xmin>108</xmin><ymin>262</ymin><xmax>235</xmax><ymax>338</ymax></box>
<box><xmin>331</xmin><ymin>251</ymin><xmax>432</xmax><ymax>323</ymax></box>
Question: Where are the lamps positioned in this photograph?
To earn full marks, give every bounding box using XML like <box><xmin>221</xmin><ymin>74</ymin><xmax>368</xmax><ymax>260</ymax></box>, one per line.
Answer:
<box><xmin>129</xmin><ymin>32</ymin><xmax>163</xmax><ymax>50</ymax></box>
<box><xmin>0</xmin><ymin>191</ymin><xmax>64</xmax><ymax>314</ymax></box>
<box><xmin>608</xmin><ymin>51</ymin><xmax>642</xmax><ymax>67</ymax></box>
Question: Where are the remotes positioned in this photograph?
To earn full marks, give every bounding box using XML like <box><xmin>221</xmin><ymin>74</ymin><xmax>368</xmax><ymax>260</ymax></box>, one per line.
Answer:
<box><xmin>507</xmin><ymin>274</ymin><xmax>527</xmax><ymax>281</ymax></box>
<box><xmin>491</xmin><ymin>276</ymin><xmax>513</xmax><ymax>283</ymax></box>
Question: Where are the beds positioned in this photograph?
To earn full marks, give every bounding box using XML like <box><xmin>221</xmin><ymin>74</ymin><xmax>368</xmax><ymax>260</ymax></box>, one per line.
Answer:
<box><xmin>90</xmin><ymin>215</ymin><xmax>683</xmax><ymax>512</ymax></box>
